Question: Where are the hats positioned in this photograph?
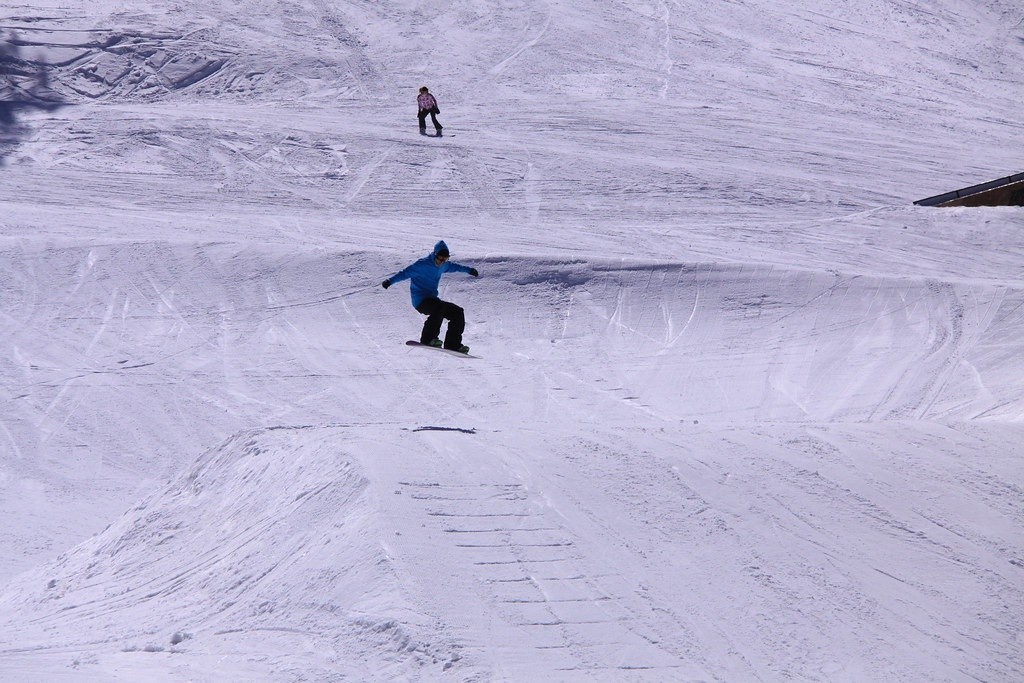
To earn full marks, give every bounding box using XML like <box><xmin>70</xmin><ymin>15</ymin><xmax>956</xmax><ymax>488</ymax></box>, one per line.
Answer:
<box><xmin>436</xmin><ymin>249</ymin><xmax>450</xmax><ymax>257</ymax></box>
<box><xmin>420</xmin><ymin>87</ymin><xmax>428</xmax><ymax>91</ymax></box>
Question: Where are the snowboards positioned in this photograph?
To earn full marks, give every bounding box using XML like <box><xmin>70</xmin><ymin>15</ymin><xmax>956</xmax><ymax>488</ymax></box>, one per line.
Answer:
<box><xmin>406</xmin><ymin>340</ymin><xmax>484</xmax><ymax>359</ymax></box>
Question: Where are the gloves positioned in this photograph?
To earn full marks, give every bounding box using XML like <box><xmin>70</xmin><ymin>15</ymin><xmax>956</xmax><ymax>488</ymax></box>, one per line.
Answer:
<box><xmin>417</xmin><ymin>113</ymin><xmax>420</xmax><ymax>118</ymax></box>
<box><xmin>435</xmin><ymin>108</ymin><xmax>440</xmax><ymax>114</ymax></box>
<box><xmin>382</xmin><ymin>279</ymin><xmax>391</xmax><ymax>290</ymax></box>
<box><xmin>469</xmin><ymin>268</ymin><xmax>478</xmax><ymax>277</ymax></box>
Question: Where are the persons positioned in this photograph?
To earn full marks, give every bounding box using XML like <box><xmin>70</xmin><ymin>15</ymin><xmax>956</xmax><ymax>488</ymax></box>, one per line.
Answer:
<box><xmin>382</xmin><ymin>240</ymin><xmax>479</xmax><ymax>353</ymax></box>
<box><xmin>417</xmin><ymin>87</ymin><xmax>443</xmax><ymax>136</ymax></box>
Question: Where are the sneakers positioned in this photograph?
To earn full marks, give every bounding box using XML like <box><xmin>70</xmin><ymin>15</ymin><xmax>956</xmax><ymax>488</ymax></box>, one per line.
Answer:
<box><xmin>420</xmin><ymin>339</ymin><xmax>443</xmax><ymax>348</ymax></box>
<box><xmin>443</xmin><ymin>344</ymin><xmax>469</xmax><ymax>354</ymax></box>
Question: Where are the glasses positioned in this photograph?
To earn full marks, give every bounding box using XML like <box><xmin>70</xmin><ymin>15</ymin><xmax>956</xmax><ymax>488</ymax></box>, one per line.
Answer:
<box><xmin>436</xmin><ymin>256</ymin><xmax>448</xmax><ymax>262</ymax></box>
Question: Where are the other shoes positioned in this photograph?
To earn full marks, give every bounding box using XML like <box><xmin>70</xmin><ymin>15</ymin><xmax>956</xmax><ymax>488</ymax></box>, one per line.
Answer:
<box><xmin>436</xmin><ymin>129</ymin><xmax>442</xmax><ymax>136</ymax></box>
<box><xmin>420</xmin><ymin>128</ymin><xmax>425</xmax><ymax>134</ymax></box>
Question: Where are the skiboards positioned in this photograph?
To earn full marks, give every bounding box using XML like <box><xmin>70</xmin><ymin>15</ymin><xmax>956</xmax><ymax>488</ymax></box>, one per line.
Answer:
<box><xmin>420</xmin><ymin>134</ymin><xmax>456</xmax><ymax>137</ymax></box>
